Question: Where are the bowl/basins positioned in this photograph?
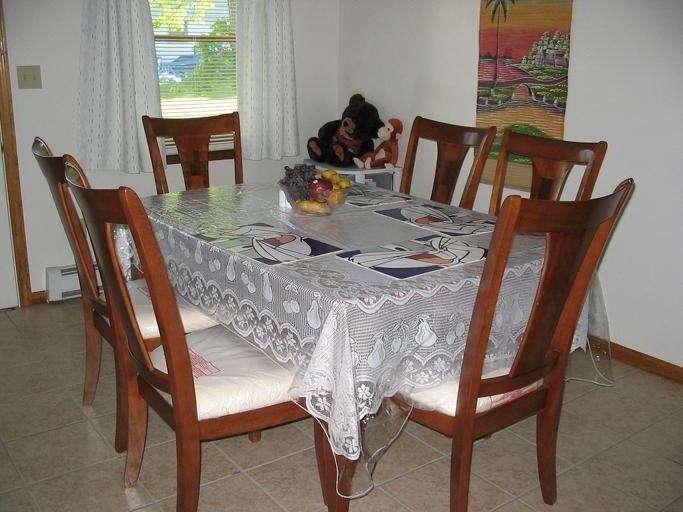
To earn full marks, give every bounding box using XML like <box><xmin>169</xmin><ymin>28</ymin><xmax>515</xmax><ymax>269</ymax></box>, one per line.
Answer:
<box><xmin>277</xmin><ymin>181</ymin><xmax>352</xmax><ymax>216</ymax></box>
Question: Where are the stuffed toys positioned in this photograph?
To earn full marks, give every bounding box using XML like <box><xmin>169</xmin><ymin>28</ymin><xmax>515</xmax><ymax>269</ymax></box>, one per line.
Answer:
<box><xmin>351</xmin><ymin>118</ymin><xmax>404</xmax><ymax>170</ymax></box>
<box><xmin>305</xmin><ymin>93</ymin><xmax>384</xmax><ymax>169</ymax></box>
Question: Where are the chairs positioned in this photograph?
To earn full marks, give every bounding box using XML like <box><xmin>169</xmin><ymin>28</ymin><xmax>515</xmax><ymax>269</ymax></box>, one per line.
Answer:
<box><xmin>142</xmin><ymin>111</ymin><xmax>243</xmax><ymax>194</ymax></box>
<box><xmin>488</xmin><ymin>129</ymin><xmax>607</xmax><ymax>221</ymax></box>
<box><xmin>60</xmin><ymin>154</ymin><xmax>332</xmax><ymax>512</ymax></box>
<box><xmin>399</xmin><ymin>115</ymin><xmax>498</xmax><ymax>210</ymax></box>
<box><xmin>30</xmin><ymin>137</ymin><xmax>261</xmax><ymax>454</ymax></box>
<box><xmin>389</xmin><ymin>177</ymin><xmax>636</xmax><ymax>512</ymax></box>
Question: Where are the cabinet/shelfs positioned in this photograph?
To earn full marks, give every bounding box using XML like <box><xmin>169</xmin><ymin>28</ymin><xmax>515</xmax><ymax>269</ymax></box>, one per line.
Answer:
<box><xmin>302</xmin><ymin>158</ymin><xmax>402</xmax><ymax>192</ymax></box>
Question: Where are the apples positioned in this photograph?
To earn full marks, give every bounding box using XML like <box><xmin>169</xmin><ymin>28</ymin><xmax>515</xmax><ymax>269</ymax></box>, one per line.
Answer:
<box><xmin>309</xmin><ymin>178</ymin><xmax>333</xmax><ymax>202</ymax></box>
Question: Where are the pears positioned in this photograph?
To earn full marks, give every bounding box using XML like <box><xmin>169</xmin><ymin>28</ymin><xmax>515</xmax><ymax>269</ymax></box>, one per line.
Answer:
<box><xmin>323</xmin><ymin>170</ymin><xmax>350</xmax><ymax>190</ymax></box>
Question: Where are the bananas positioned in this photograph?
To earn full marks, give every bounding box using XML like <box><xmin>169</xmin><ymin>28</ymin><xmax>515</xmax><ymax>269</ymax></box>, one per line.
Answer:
<box><xmin>298</xmin><ymin>201</ymin><xmax>330</xmax><ymax>214</ymax></box>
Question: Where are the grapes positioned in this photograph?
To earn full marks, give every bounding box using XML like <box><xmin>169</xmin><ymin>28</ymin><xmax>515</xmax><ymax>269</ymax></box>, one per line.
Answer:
<box><xmin>280</xmin><ymin>164</ymin><xmax>316</xmax><ymax>200</ymax></box>
